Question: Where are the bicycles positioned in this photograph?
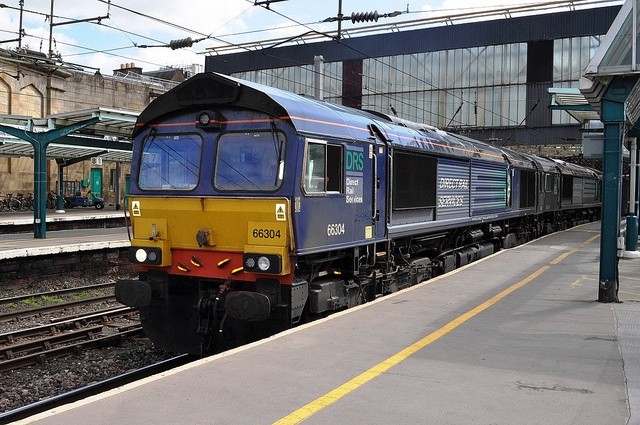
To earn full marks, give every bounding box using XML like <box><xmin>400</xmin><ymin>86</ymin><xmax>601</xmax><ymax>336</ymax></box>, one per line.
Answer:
<box><xmin>0</xmin><ymin>190</ymin><xmax>58</xmax><ymax>212</ymax></box>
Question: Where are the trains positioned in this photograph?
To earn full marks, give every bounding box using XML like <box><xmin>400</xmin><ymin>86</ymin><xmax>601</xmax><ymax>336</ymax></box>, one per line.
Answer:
<box><xmin>115</xmin><ymin>71</ymin><xmax>603</xmax><ymax>358</ymax></box>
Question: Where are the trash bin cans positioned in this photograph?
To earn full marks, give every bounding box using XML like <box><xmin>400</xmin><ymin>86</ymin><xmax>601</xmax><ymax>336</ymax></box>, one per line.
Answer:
<box><xmin>56</xmin><ymin>195</ymin><xmax>64</xmax><ymax>210</ymax></box>
